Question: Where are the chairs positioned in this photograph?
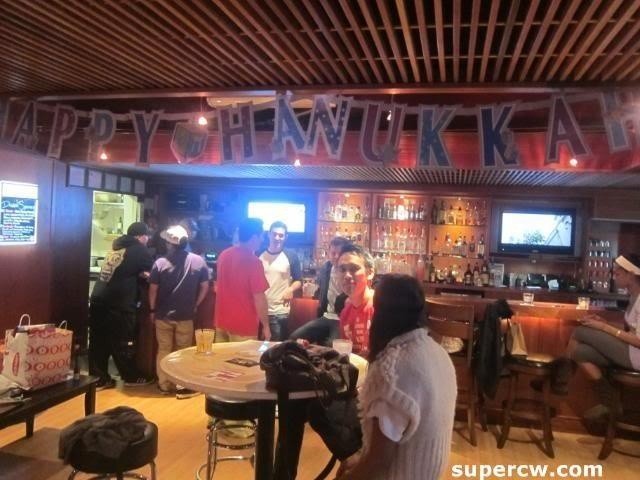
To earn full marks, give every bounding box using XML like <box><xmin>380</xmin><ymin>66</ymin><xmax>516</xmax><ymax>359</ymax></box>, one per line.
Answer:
<box><xmin>423</xmin><ymin>298</ymin><xmax>489</xmax><ymax>449</ymax></box>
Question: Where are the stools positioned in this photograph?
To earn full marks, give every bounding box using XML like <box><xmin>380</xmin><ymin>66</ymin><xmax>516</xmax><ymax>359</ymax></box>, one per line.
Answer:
<box><xmin>598</xmin><ymin>366</ymin><xmax>640</xmax><ymax>460</ymax></box>
<box><xmin>196</xmin><ymin>394</ymin><xmax>259</xmax><ymax>479</ymax></box>
<box><xmin>66</xmin><ymin>419</ymin><xmax>159</xmax><ymax>480</ymax></box>
<box><xmin>498</xmin><ymin>350</ymin><xmax>557</xmax><ymax>457</ymax></box>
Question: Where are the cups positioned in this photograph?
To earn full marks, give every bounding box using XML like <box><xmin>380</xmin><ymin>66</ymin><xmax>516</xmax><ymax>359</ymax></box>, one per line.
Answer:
<box><xmin>578</xmin><ymin>296</ymin><xmax>590</xmax><ymax>310</ymax></box>
<box><xmin>196</xmin><ymin>331</ymin><xmax>214</xmax><ymax>354</ymax></box>
<box><xmin>334</xmin><ymin>339</ymin><xmax>351</xmax><ymax>357</ymax></box>
<box><xmin>523</xmin><ymin>294</ymin><xmax>533</xmax><ymax>304</ymax></box>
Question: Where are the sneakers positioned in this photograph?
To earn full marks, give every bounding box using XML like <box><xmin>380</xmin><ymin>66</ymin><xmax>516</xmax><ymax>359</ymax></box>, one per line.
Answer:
<box><xmin>529</xmin><ymin>378</ymin><xmax>573</xmax><ymax>397</ymax></box>
<box><xmin>123</xmin><ymin>376</ymin><xmax>157</xmax><ymax>388</ymax></box>
<box><xmin>175</xmin><ymin>386</ymin><xmax>202</xmax><ymax>399</ymax></box>
<box><xmin>154</xmin><ymin>385</ymin><xmax>173</xmax><ymax>396</ymax></box>
<box><xmin>95</xmin><ymin>377</ymin><xmax>118</xmax><ymax>392</ymax></box>
<box><xmin>582</xmin><ymin>403</ymin><xmax>624</xmax><ymax>421</ymax></box>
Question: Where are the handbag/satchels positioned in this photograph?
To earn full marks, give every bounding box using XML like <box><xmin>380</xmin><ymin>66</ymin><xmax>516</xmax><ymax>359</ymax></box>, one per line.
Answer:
<box><xmin>1</xmin><ymin>313</ymin><xmax>74</xmax><ymax>392</ymax></box>
<box><xmin>505</xmin><ymin>311</ymin><xmax>529</xmax><ymax>357</ymax></box>
<box><xmin>259</xmin><ymin>340</ymin><xmax>350</xmax><ymax>411</ymax></box>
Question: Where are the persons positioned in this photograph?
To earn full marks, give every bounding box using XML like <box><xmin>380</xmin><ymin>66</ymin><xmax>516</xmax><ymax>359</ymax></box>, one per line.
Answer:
<box><xmin>149</xmin><ymin>225</ymin><xmax>209</xmax><ymax>398</ymax></box>
<box><xmin>255</xmin><ymin>221</ymin><xmax>303</xmax><ymax>417</ymax></box>
<box><xmin>88</xmin><ymin>221</ymin><xmax>155</xmax><ymax>391</ymax></box>
<box><xmin>529</xmin><ymin>250</ymin><xmax>640</xmax><ymax>423</ymax></box>
<box><xmin>213</xmin><ymin>217</ymin><xmax>272</xmax><ymax>344</ymax></box>
<box><xmin>332</xmin><ymin>275</ymin><xmax>459</xmax><ymax>480</ymax></box>
<box><xmin>307</xmin><ymin>243</ymin><xmax>376</xmax><ymax>459</ymax></box>
<box><xmin>288</xmin><ymin>237</ymin><xmax>349</xmax><ymax>347</ymax></box>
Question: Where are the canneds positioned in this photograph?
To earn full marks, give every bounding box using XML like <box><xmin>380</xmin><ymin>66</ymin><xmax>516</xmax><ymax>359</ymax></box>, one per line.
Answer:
<box><xmin>586</xmin><ymin>237</ymin><xmax>612</xmax><ymax>279</ymax></box>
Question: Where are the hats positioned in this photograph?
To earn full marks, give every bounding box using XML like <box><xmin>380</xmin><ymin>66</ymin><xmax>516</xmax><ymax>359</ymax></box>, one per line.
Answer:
<box><xmin>127</xmin><ymin>220</ymin><xmax>157</xmax><ymax>237</ymax></box>
<box><xmin>160</xmin><ymin>224</ymin><xmax>189</xmax><ymax>247</ymax></box>
<box><xmin>373</xmin><ymin>273</ymin><xmax>425</xmax><ymax>313</ymax></box>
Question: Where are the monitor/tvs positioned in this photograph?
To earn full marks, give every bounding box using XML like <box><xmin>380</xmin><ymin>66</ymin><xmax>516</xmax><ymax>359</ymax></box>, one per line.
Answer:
<box><xmin>246</xmin><ymin>201</ymin><xmax>306</xmax><ymax>233</ymax></box>
<box><xmin>496</xmin><ymin>206</ymin><xmax>575</xmax><ymax>253</ymax></box>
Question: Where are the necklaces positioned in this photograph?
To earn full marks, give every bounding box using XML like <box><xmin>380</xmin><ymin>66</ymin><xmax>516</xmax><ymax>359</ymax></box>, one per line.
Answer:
<box><xmin>266</xmin><ymin>247</ymin><xmax>283</xmax><ymax>254</ymax></box>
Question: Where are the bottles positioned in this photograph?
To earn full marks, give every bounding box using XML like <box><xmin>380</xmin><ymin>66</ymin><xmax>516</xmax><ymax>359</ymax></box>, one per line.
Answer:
<box><xmin>374</xmin><ymin>223</ymin><xmax>427</xmax><ymax>253</ymax></box>
<box><xmin>432</xmin><ymin>230</ymin><xmax>485</xmax><ymax>258</ymax></box>
<box><xmin>319</xmin><ymin>195</ymin><xmax>370</xmax><ymax>221</ymax></box>
<box><xmin>430</xmin><ymin>198</ymin><xmax>482</xmax><ymax>226</ymax></box>
<box><xmin>373</xmin><ymin>255</ymin><xmax>426</xmax><ymax>282</ymax></box>
<box><xmin>377</xmin><ymin>198</ymin><xmax>428</xmax><ymax>221</ymax></box>
<box><xmin>428</xmin><ymin>259</ymin><xmax>490</xmax><ymax>286</ymax></box>
<box><xmin>319</xmin><ymin>225</ymin><xmax>369</xmax><ymax>249</ymax></box>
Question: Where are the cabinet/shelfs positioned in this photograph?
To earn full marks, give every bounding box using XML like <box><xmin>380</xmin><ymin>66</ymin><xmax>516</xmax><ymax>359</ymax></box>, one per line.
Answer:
<box><xmin>310</xmin><ymin>188</ymin><xmax>495</xmax><ymax>288</ymax></box>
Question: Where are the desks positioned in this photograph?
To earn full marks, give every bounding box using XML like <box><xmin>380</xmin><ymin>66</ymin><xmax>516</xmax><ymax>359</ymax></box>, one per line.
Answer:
<box><xmin>1</xmin><ymin>373</ymin><xmax>99</xmax><ymax>446</ymax></box>
<box><xmin>159</xmin><ymin>340</ymin><xmax>373</xmax><ymax>480</ymax></box>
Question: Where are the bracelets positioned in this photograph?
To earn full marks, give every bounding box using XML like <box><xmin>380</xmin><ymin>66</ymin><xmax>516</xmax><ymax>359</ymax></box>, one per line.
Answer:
<box><xmin>615</xmin><ymin>329</ymin><xmax>620</xmax><ymax>337</ymax></box>
<box><xmin>149</xmin><ymin>308</ymin><xmax>156</xmax><ymax>313</ymax></box>
